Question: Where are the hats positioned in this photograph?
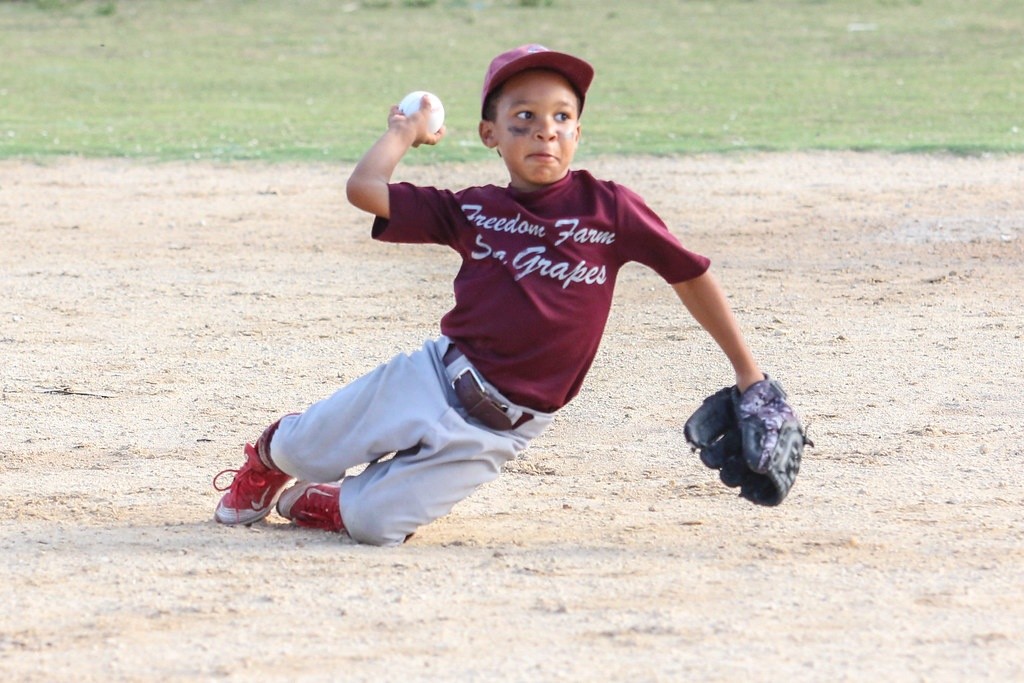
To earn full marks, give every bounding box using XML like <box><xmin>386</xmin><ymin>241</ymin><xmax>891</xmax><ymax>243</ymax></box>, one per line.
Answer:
<box><xmin>481</xmin><ymin>44</ymin><xmax>595</xmax><ymax>120</ymax></box>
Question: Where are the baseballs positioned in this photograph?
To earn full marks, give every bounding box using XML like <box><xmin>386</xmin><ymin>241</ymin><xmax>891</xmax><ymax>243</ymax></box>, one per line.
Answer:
<box><xmin>400</xmin><ymin>92</ymin><xmax>447</xmax><ymax>134</ymax></box>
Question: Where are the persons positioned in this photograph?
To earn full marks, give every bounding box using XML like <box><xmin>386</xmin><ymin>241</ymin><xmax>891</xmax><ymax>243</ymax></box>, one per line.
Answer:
<box><xmin>211</xmin><ymin>41</ymin><xmax>815</xmax><ymax>550</ymax></box>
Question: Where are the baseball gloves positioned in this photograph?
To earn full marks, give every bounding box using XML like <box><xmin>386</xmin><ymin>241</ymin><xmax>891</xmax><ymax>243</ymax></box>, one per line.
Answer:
<box><xmin>682</xmin><ymin>370</ymin><xmax>811</xmax><ymax>508</ymax></box>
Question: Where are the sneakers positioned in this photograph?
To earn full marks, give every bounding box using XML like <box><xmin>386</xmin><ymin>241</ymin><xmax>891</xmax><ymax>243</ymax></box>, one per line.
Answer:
<box><xmin>276</xmin><ymin>481</ymin><xmax>347</xmax><ymax>534</ymax></box>
<box><xmin>213</xmin><ymin>414</ymin><xmax>303</xmax><ymax>526</ymax></box>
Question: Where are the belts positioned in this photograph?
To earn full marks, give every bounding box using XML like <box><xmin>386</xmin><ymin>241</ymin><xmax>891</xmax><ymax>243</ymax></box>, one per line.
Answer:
<box><xmin>443</xmin><ymin>344</ymin><xmax>533</xmax><ymax>432</ymax></box>
